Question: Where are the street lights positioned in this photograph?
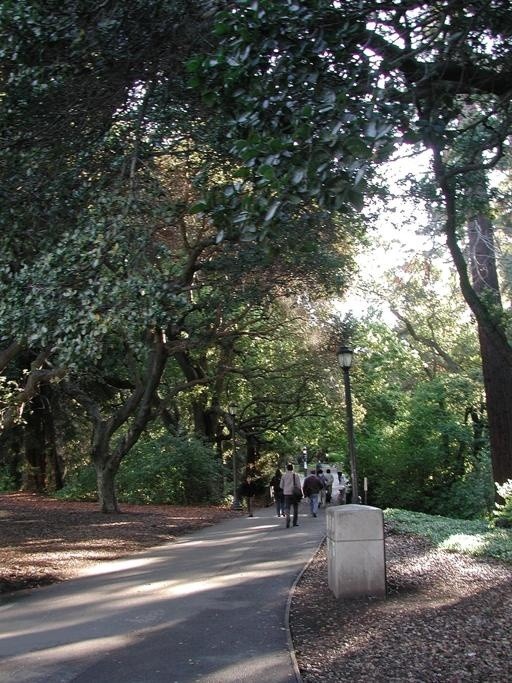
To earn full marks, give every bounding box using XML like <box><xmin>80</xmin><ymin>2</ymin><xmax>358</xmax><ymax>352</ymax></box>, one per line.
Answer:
<box><xmin>303</xmin><ymin>446</ymin><xmax>310</xmax><ymax>476</ymax></box>
<box><xmin>225</xmin><ymin>401</ymin><xmax>244</xmax><ymax>511</ymax></box>
<box><xmin>336</xmin><ymin>344</ymin><xmax>362</xmax><ymax>505</ymax></box>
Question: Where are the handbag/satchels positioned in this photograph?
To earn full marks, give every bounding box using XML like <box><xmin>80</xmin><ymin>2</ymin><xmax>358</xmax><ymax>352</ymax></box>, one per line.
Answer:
<box><xmin>292</xmin><ymin>486</ymin><xmax>303</xmax><ymax>502</ymax></box>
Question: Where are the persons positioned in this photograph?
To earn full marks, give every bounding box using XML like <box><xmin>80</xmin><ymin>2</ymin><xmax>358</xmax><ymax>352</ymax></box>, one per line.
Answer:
<box><xmin>242</xmin><ymin>459</ymin><xmax>348</xmax><ymax>528</ymax></box>
